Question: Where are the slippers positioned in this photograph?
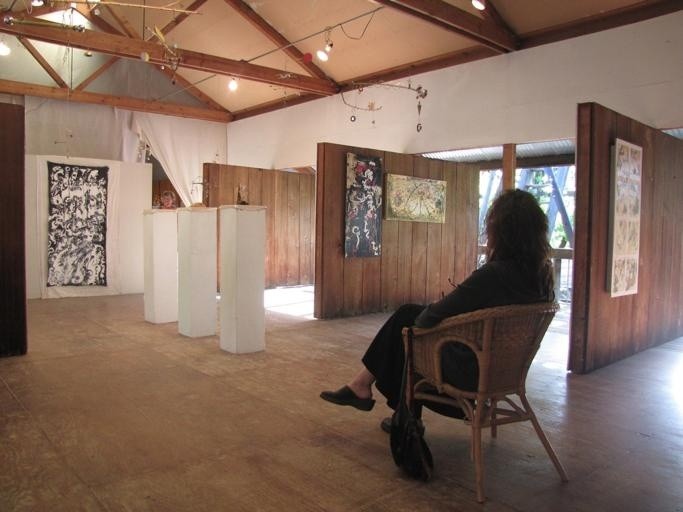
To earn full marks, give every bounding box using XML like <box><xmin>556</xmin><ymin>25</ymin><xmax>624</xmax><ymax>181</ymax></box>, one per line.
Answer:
<box><xmin>381</xmin><ymin>417</ymin><xmax>425</xmax><ymax>436</ymax></box>
<box><xmin>320</xmin><ymin>385</ymin><xmax>376</xmax><ymax>411</ymax></box>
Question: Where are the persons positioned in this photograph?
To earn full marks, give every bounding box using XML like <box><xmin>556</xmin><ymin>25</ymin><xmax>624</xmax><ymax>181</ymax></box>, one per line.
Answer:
<box><xmin>159</xmin><ymin>191</ymin><xmax>177</xmax><ymax>210</ymax></box>
<box><xmin>317</xmin><ymin>188</ymin><xmax>554</xmax><ymax>435</ymax></box>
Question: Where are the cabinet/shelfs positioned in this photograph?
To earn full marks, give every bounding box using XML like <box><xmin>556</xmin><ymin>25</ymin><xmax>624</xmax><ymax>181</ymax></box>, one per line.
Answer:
<box><xmin>175</xmin><ymin>205</ymin><xmax>216</xmax><ymax>338</ymax></box>
<box><xmin>145</xmin><ymin>208</ymin><xmax>178</xmax><ymax>325</ymax></box>
<box><xmin>218</xmin><ymin>201</ymin><xmax>269</xmax><ymax>355</ymax></box>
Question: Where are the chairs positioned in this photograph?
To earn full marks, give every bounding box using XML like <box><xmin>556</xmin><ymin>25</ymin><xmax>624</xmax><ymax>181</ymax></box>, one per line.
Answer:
<box><xmin>397</xmin><ymin>298</ymin><xmax>570</xmax><ymax>505</ymax></box>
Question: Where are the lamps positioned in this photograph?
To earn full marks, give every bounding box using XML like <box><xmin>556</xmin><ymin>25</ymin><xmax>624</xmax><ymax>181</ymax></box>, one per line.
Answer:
<box><xmin>27</xmin><ymin>0</ymin><xmax>105</xmax><ymax>19</ymax></box>
<box><xmin>314</xmin><ymin>25</ymin><xmax>336</xmax><ymax>61</ymax></box>
<box><xmin>226</xmin><ymin>72</ymin><xmax>241</xmax><ymax>90</ymax></box>
